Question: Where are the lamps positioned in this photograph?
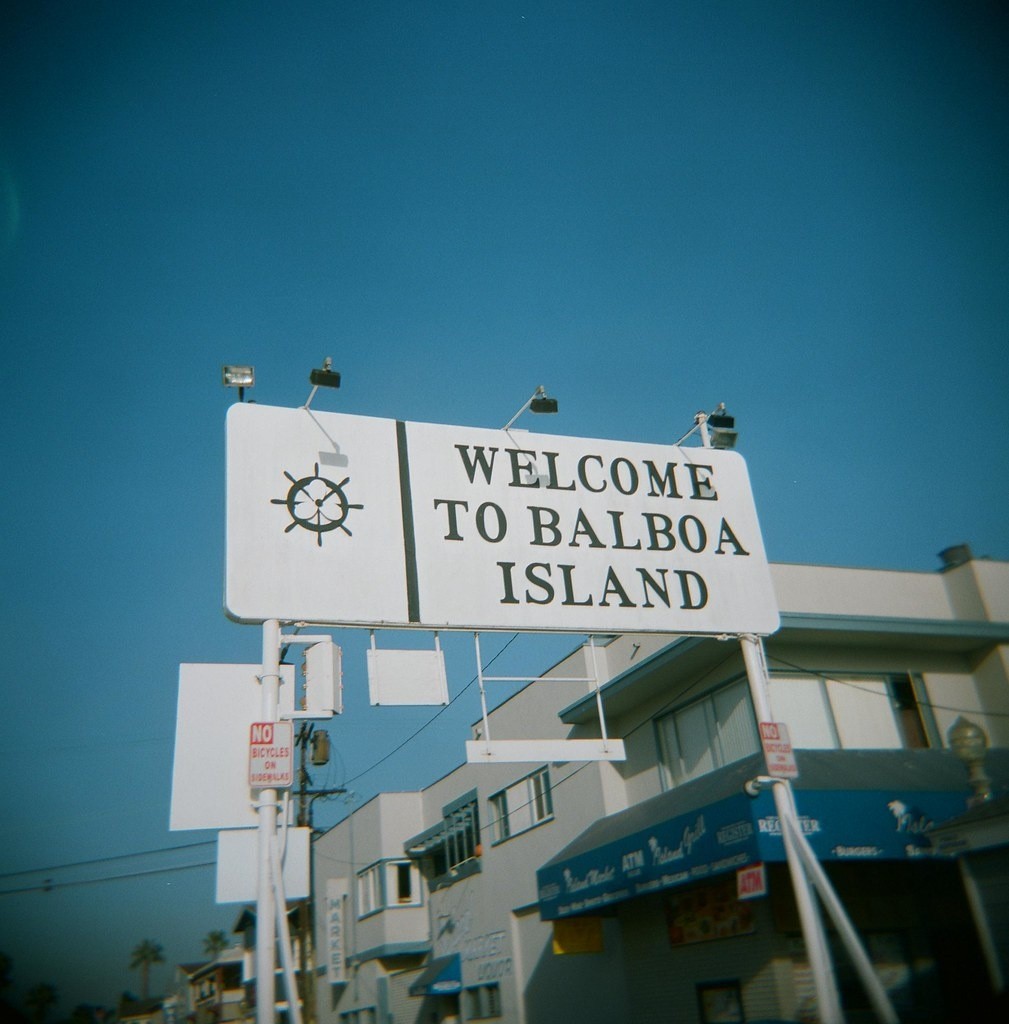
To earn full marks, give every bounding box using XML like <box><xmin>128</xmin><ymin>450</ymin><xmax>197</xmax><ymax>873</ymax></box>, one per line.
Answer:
<box><xmin>305</xmin><ymin>356</ymin><xmax>341</xmax><ymax>410</ymax></box>
<box><xmin>675</xmin><ymin>402</ymin><xmax>738</xmax><ymax>450</ymax></box>
<box><xmin>224</xmin><ymin>366</ymin><xmax>253</xmax><ymax>402</ymax></box>
<box><xmin>505</xmin><ymin>385</ymin><xmax>558</xmax><ymax>429</ymax></box>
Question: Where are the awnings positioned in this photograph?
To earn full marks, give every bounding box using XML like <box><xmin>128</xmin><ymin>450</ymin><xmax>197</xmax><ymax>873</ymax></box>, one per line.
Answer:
<box><xmin>408</xmin><ymin>953</ymin><xmax>461</xmax><ymax>996</ymax></box>
<box><xmin>535</xmin><ymin>749</ymin><xmax>1009</xmax><ymax>921</ymax></box>
